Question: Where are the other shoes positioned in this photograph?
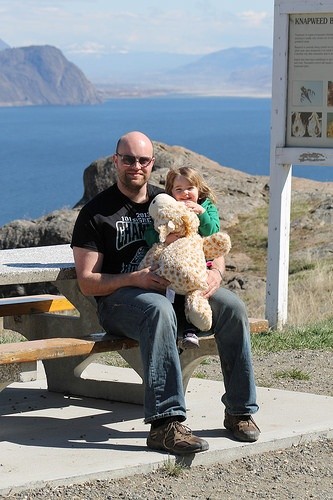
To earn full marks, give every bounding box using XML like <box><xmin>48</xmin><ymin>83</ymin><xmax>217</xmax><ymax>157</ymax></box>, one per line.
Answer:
<box><xmin>182</xmin><ymin>327</ymin><xmax>199</xmax><ymax>348</ymax></box>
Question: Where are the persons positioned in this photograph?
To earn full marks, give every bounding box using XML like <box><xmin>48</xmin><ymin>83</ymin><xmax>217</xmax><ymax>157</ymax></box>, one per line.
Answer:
<box><xmin>70</xmin><ymin>130</ymin><xmax>263</xmax><ymax>457</ymax></box>
<box><xmin>143</xmin><ymin>166</ymin><xmax>221</xmax><ymax>352</ymax></box>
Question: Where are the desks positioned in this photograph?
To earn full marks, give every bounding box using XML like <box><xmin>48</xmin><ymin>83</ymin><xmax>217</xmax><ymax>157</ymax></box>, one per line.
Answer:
<box><xmin>0</xmin><ymin>244</ymin><xmax>105</xmax><ymax>336</ymax></box>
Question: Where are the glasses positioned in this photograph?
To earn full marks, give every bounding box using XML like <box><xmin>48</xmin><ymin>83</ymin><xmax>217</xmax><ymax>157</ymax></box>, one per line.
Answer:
<box><xmin>117</xmin><ymin>153</ymin><xmax>152</xmax><ymax>167</ymax></box>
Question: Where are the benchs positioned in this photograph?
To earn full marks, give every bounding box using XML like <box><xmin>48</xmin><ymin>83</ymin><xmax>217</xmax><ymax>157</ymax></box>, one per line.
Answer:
<box><xmin>0</xmin><ymin>291</ymin><xmax>270</xmax><ymax>407</ymax></box>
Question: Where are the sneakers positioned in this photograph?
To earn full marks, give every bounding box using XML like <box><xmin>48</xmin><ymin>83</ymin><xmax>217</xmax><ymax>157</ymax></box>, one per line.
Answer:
<box><xmin>147</xmin><ymin>417</ymin><xmax>209</xmax><ymax>455</ymax></box>
<box><xmin>224</xmin><ymin>408</ymin><xmax>261</xmax><ymax>442</ymax></box>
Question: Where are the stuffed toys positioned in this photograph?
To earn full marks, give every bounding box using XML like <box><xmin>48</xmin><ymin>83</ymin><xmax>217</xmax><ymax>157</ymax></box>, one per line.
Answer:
<box><xmin>137</xmin><ymin>192</ymin><xmax>232</xmax><ymax>332</ymax></box>
